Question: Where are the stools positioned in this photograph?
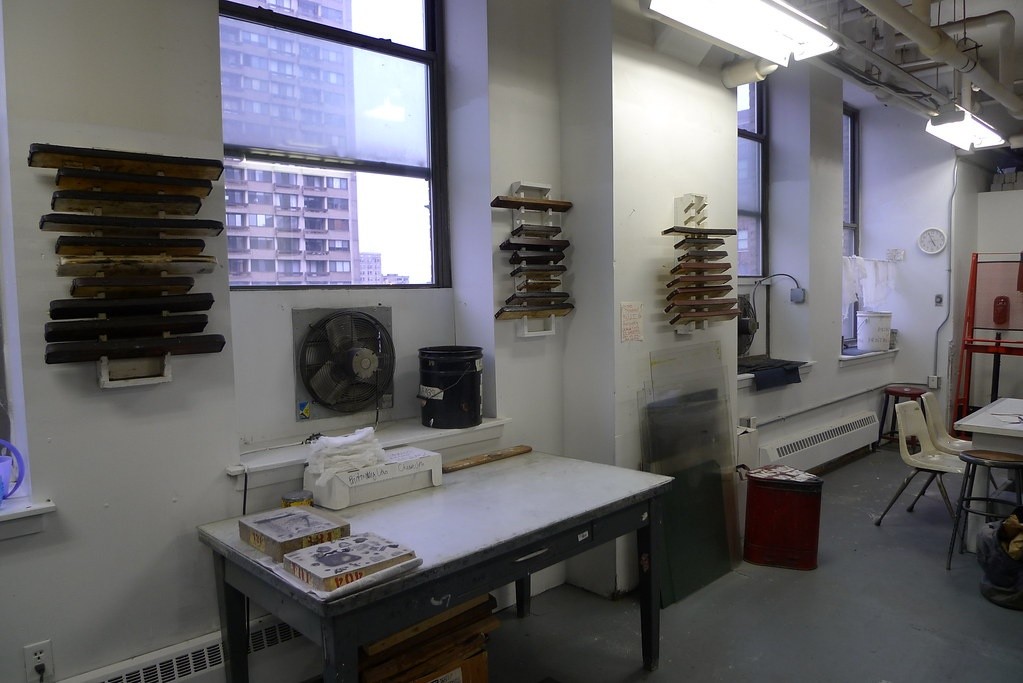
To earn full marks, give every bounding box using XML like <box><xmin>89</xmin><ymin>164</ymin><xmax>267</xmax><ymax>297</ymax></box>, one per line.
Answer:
<box><xmin>944</xmin><ymin>450</ymin><xmax>1023</xmax><ymax>570</ymax></box>
<box><xmin>876</xmin><ymin>386</ymin><xmax>928</xmax><ymax>453</ymax></box>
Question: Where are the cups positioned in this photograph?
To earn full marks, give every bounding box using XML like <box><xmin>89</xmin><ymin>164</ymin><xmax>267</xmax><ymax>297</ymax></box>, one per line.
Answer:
<box><xmin>0</xmin><ymin>455</ymin><xmax>13</xmax><ymax>507</ymax></box>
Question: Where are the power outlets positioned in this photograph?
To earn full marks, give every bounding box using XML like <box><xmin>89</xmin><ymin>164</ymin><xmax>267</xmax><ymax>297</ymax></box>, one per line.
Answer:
<box><xmin>24</xmin><ymin>638</ymin><xmax>54</xmax><ymax>682</ymax></box>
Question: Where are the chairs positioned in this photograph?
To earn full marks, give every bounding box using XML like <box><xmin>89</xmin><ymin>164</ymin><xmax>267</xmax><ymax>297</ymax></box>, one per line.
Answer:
<box><xmin>874</xmin><ymin>400</ymin><xmax>972</xmax><ymax>546</ymax></box>
<box><xmin>907</xmin><ymin>392</ymin><xmax>998</xmax><ymax>517</ymax></box>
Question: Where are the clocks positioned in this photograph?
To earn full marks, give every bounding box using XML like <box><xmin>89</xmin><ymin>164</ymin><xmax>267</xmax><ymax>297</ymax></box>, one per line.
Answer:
<box><xmin>918</xmin><ymin>227</ymin><xmax>947</xmax><ymax>255</ymax></box>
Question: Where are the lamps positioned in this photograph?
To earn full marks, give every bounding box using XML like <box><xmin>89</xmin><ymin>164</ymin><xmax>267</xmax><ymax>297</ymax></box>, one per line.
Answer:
<box><xmin>925</xmin><ymin>102</ymin><xmax>1013</xmax><ymax>154</ymax></box>
<box><xmin>641</xmin><ymin>0</ymin><xmax>849</xmax><ymax>69</ymax></box>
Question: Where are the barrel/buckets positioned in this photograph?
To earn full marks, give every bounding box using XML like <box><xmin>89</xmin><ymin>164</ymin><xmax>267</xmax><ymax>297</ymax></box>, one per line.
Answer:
<box><xmin>856</xmin><ymin>310</ymin><xmax>893</xmax><ymax>351</ymax></box>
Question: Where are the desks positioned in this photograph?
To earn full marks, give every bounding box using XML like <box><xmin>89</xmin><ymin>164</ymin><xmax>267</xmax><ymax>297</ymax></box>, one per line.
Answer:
<box><xmin>197</xmin><ymin>445</ymin><xmax>674</xmax><ymax>683</ymax></box>
<box><xmin>952</xmin><ymin>397</ymin><xmax>1023</xmax><ymax>553</ymax></box>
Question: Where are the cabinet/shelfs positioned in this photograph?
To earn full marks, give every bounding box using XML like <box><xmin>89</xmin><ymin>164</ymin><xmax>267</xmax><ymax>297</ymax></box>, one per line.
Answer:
<box><xmin>27</xmin><ymin>143</ymin><xmax>229</xmax><ymax>391</ymax></box>
<box><xmin>487</xmin><ymin>180</ymin><xmax>576</xmax><ymax>339</ymax></box>
<box><xmin>659</xmin><ymin>193</ymin><xmax>743</xmax><ymax>339</ymax></box>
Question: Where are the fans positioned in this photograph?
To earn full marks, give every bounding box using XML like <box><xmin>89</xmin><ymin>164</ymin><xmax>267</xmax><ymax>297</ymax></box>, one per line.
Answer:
<box><xmin>290</xmin><ymin>304</ymin><xmax>394</xmax><ymax>423</ymax></box>
<box><xmin>736</xmin><ymin>296</ymin><xmax>758</xmax><ymax>358</ymax></box>
